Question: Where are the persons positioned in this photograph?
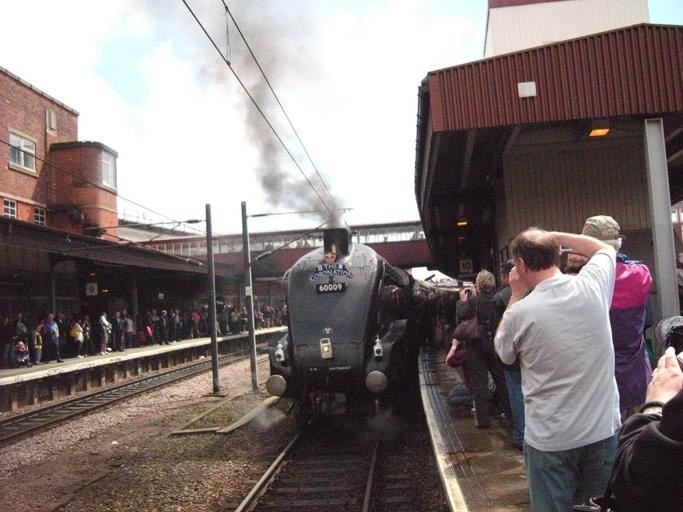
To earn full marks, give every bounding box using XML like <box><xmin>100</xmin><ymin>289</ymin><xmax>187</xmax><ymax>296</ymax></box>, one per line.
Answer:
<box><xmin>0</xmin><ymin>302</ymin><xmax>288</xmax><ymax>368</ymax></box>
<box><xmin>494</xmin><ymin>226</ymin><xmax>622</xmax><ymax>512</ymax></box>
<box><xmin>445</xmin><ymin>258</ymin><xmax>525</xmax><ymax>450</ymax></box>
<box><xmin>579</xmin><ymin>216</ymin><xmax>652</xmax><ymax>422</ymax></box>
<box><xmin>416</xmin><ymin>290</ymin><xmax>457</xmax><ymax>351</ymax></box>
<box><xmin>602</xmin><ymin>346</ymin><xmax>683</xmax><ymax>512</ymax></box>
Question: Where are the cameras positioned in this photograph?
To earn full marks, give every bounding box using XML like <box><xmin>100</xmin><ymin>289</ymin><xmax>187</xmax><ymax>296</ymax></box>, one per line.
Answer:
<box><xmin>465</xmin><ymin>285</ymin><xmax>471</xmax><ymax>290</ymax></box>
<box><xmin>560</xmin><ymin>245</ymin><xmax>572</xmax><ymax>251</ymax></box>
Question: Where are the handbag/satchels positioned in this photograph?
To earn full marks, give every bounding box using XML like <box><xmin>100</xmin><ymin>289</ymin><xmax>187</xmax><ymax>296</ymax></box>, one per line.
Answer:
<box><xmin>453</xmin><ymin>318</ymin><xmax>481</xmax><ymax>342</ymax></box>
<box><xmin>70</xmin><ymin>328</ymin><xmax>80</xmax><ymax>338</ymax></box>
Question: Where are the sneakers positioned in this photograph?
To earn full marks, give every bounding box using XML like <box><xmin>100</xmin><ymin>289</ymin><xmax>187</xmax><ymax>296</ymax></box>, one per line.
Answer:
<box><xmin>100</xmin><ymin>352</ymin><xmax>108</xmax><ymax>355</ymax></box>
<box><xmin>57</xmin><ymin>359</ymin><xmax>64</xmax><ymax>363</ymax></box>
<box><xmin>76</xmin><ymin>353</ymin><xmax>87</xmax><ymax>358</ymax></box>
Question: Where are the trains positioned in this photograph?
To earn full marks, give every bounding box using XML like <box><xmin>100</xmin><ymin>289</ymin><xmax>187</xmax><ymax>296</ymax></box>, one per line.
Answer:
<box><xmin>266</xmin><ymin>228</ymin><xmax>440</xmax><ymax>425</ymax></box>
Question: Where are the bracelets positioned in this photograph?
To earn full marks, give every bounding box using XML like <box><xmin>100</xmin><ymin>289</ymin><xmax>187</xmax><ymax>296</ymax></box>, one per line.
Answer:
<box><xmin>640</xmin><ymin>400</ymin><xmax>665</xmax><ymax>415</ymax></box>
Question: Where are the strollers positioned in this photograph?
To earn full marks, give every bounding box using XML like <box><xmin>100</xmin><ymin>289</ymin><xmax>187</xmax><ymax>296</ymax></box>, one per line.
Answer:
<box><xmin>12</xmin><ymin>335</ymin><xmax>32</xmax><ymax>368</ymax></box>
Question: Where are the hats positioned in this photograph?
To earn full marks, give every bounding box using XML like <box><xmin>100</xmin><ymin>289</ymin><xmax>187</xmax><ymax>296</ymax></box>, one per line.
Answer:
<box><xmin>581</xmin><ymin>215</ymin><xmax>620</xmax><ymax>239</ymax></box>
